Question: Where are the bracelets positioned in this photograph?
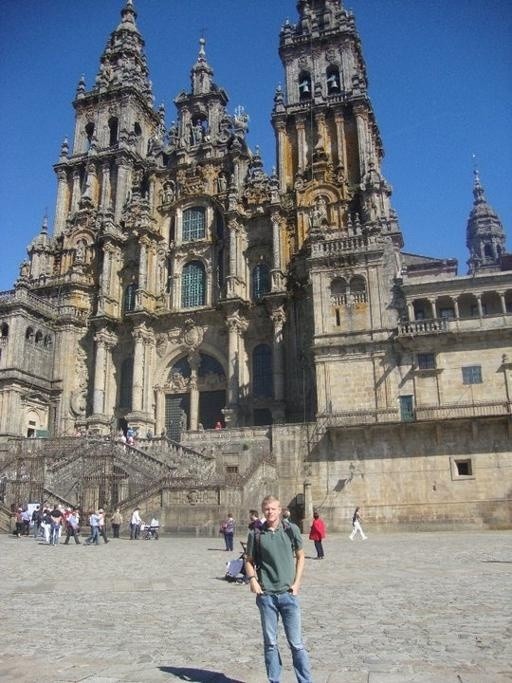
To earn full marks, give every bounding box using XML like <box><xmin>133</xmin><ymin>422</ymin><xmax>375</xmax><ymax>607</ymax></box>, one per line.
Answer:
<box><xmin>247</xmin><ymin>575</ymin><xmax>255</xmax><ymax>582</ymax></box>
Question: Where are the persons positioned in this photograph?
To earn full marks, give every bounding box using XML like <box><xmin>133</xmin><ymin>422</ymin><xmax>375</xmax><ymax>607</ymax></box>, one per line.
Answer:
<box><xmin>247</xmin><ymin>508</ymin><xmax>263</xmax><ymax>531</ymax></box>
<box><xmin>158</xmin><ymin>183</ymin><xmax>174</xmax><ymax>204</ymax></box>
<box><xmin>217</xmin><ymin>171</ymin><xmax>227</xmax><ymax>193</ymax></box>
<box><xmin>308</xmin><ymin>206</ymin><xmax>322</xmax><ymax>228</ymax></box>
<box><xmin>127</xmin><ymin>433</ymin><xmax>134</xmax><ymax>446</ymax></box>
<box><xmin>318</xmin><ymin>195</ymin><xmax>328</xmax><ymax>220</ymax></box>
<box><xmin>221</xmin><ymin>512</ymin><xmax>236</xmax><ymax>551</ymax></box>
<box><xmin>348</xmin><ymin>506</ymin><xmax>369</xmax><ymax>541</ymax></box>
<box><xmin>146</xmin><ymin>427</ymin><xmax>153</xmax><ymax>437</ymax></box>
<box><xmin>12</xmin><ymin>500</ymin><xmax>159</xmax><ymax>544</ymax></box>
<box><xmin>244</xmin><ymin>493</ymin><xmax>313</xmax><ymax>683</ymax></box>
<box><xmin>215</xmin><ymin>420</ymin><xmax>222</xmax><ymax>429</ymax></box>
<box><xmin>117</xmin><ymin>431</ymin><xmax>126</xmax><ymax>451</ymax></box>
<box><xmin>126</xmin><ymin>425</ymin><xmax>134</xmax><ymax>438</ymax></box>
<box><xmin>188</xmin><ymin>118</ymin><xmax>205</xmax><ymax>145</ymax></box>
<box><xmin>309</xmin><ymin>511</ymin><xmax>327</xmax><ymax>560</ymax></box>
<box><xmin>281</xmin><ymin>510</ymin><xmax>291</xmax><ymax>523</ymax></box>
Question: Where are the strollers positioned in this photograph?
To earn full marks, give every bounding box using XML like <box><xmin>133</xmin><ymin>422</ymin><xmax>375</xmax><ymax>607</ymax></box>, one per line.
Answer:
<box><xmin>140</xmin><ymin>521</ymin><xmax>160</xmax><ymax>539</ymax></box>
<box><xmin>224</xmin><ymin>541</ymin><xmax>250</xmax><ymax>584</ymax></box>
